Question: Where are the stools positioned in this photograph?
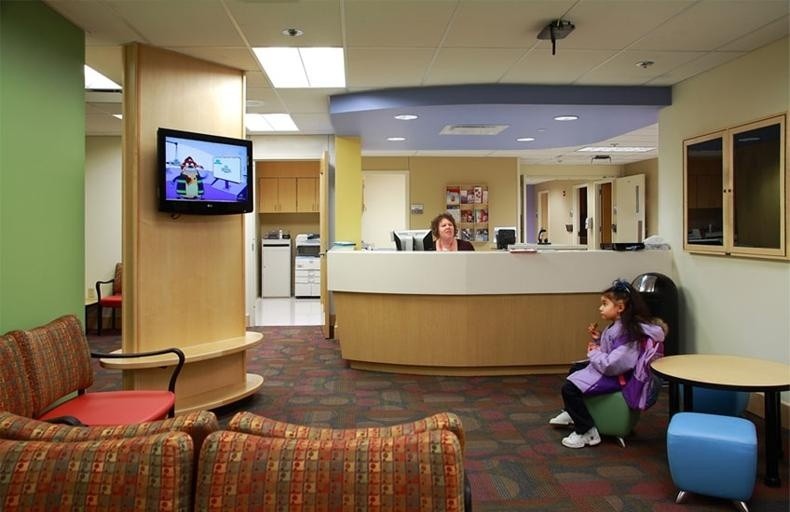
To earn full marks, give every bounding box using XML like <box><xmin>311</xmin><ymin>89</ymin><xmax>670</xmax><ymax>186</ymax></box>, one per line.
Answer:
<box><xmin>583</xmin><ymin>385</ymin><xmax>637</xmax><ymax>449</ymax></box>
<box><xmin>677</xmin><ymin>380</ymin><xmax>752</xmax><ymax>418</ymax></box>
<box><xmin>665</xmin><ymin>409</ymin><xmax>763</xmax><ymax>512</ymax></box>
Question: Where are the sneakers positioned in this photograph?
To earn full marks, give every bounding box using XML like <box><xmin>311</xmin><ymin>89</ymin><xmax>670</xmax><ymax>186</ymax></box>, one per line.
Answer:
<box><xmin>562</xmin><ymin>427</ymin><xmax>602</xmax><ymax>449</ymax></box>
<box><xmin>548</xmin><ymin>410</ymin><xmax>575</xmax><ymax>425</ymax></box>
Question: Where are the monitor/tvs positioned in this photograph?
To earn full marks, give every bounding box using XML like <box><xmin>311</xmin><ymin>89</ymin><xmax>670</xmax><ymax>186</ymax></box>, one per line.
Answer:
<box><xmin>155</xmin><ymin>127</ymin><xmax>254</xmax><ymax>214</ymax></box>
<box><xmin>392</xmin><ymin>230</ymin><xmax>433</xmax><ymax>251</ymax></box>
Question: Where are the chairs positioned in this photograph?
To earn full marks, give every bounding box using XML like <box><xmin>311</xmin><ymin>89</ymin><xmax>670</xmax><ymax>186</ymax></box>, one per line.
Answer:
<box><xmin>0</xmin><ymin>311</ymin><xmax>187</xmax><ymax>430</ymax></box>
<box><xmin>92</xmin><ymin>259</ymin><xmax>123</xmax><ymax>337</ymax></box>
<box><xmin>1</xmin><ymin>412</ymin><xmax>219</xmax><ymax>441</ymax></box>
<box><xmin>1</xmin><ymin>430</ymin><xmax>192</xmax><ymax>511</ymax></box>
<box><xmin>194</xmin><ymin>430</ymin><xmax>467</xmax><ymax>512</ymax></box>
<box><xmin>229</xmin><ymin>411</ymin><xmax>472</xmax><ymax>512</ymax></box>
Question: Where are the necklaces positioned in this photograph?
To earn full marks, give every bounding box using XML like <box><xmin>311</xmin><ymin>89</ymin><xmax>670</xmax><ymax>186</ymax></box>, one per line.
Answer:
<box><xmin>438</xmin><ymin>238</ymin><xmax>454</xmax><ymax>251</ymax></box>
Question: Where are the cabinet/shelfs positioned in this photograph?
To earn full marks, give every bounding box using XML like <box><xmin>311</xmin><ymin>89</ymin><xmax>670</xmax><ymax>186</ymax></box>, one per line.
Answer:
<box><xmin>259</xmin><ymin>177</ymin><xmax>297</xmax><ymax>214</ymax></box>
<box><xmin>100</xmin><ymin>332</ymin><xmax>266</xmax><ymax>423</ymax></box>
<box><xmin>297</xmin><ymin>178</ymin><xmax>320</xmax><ymax>214</ymax></box>
<box><xmin>446</xmin><ymin>182</ymin><xmax>490</xmax><ymax>244</ymax></box>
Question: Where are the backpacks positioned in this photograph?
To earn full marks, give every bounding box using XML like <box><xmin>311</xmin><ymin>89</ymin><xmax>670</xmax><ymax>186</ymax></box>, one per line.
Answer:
<box><xmin>625</xmin><ymin>337</ymin><xmax>664</xmax><ymax>410</ymax></box>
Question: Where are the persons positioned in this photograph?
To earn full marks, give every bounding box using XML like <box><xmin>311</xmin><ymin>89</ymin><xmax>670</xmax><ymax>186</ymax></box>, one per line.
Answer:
<box><xmin>548</xmin><ymin>279</ymin><xmax>648</xmax><ymax>447</ymax></box>
<box><xmin>427</xmin><ymin>212</ymin><xmax>475</xmax><ymax>252</ymax></box>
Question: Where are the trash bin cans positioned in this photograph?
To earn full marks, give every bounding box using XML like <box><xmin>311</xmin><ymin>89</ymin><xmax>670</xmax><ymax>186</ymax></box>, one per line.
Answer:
<box><xmin>630</xmin><ymin>273</ymin><xmax>679</xmax><ymax>386</ymax></box>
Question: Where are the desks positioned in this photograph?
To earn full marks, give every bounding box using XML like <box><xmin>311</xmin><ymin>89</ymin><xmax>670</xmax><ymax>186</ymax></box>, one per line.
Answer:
<box><xmin>648</xmin><ymin>352</ymin><xmax>790</xmax><ymax>488</ymax></box>
<box><xmin>85</xmin><ymin>283</ymin><xmax>104</xmax><ymax>336</ymax></box>
